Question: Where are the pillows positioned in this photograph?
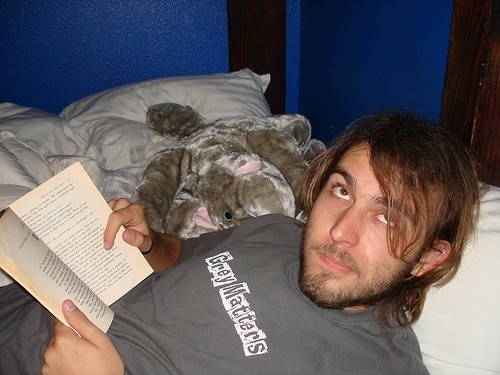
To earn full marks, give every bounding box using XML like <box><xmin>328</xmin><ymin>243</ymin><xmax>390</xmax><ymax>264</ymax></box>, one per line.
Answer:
<box><xmin>0</xmin><ymin>131</ymin><xmax>56</xmax><ymax>287</ymax></box>
<box><xmin>62</xmin><ymin>69</ymin><xmax>271</xmax><ymax>168</ymax></box>
<box><xmin>1</xmin><ymin>101</ymin><xmax>105</xmax><ymax>193</ymax></box>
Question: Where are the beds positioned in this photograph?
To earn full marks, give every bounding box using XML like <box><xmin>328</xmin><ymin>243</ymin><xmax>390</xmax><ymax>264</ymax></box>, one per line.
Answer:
<box><xmin>406</xmin><ymin>178</ymin><xmax>500</xmax><ymax>375</ymax></box>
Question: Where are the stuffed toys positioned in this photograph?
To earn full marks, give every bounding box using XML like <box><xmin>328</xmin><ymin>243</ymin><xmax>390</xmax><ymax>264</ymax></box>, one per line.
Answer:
<box><xmin>134</xmin><ymin>100</ymin><xmax>327</xmax><ymax>239</ymax></box>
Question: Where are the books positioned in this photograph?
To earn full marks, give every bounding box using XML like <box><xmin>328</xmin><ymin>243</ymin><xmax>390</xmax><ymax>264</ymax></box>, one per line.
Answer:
<box><xmin>0</xmin><ymin>160</ymin><xmax>154</xmax><ymax>344</ymax></box>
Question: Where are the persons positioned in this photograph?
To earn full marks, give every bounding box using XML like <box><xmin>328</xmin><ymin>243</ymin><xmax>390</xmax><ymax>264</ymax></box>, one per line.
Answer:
<box><xmin>0</xmin><ymin>107</ymin><xmax>483</xmax><ymax>375</ymax></box>
